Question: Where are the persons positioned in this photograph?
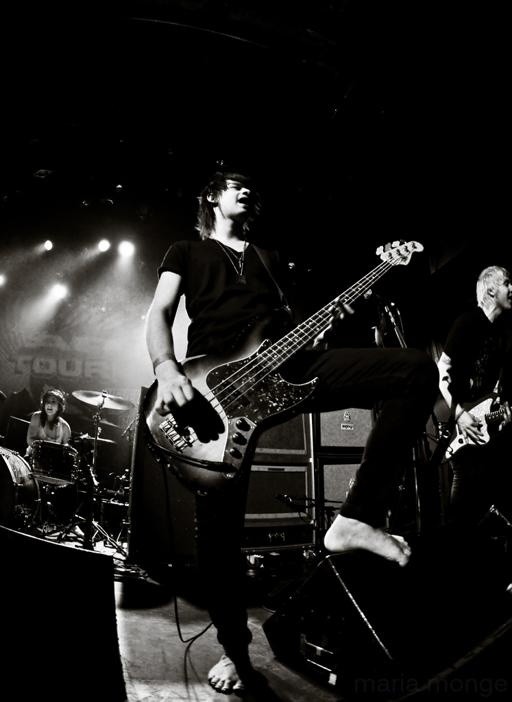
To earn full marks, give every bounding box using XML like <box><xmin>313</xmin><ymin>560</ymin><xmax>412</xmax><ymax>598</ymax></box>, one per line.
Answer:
<box><xmin>435</xmin><ymin>263</ymin><xmax>512</xmax><ymax>547</ymax></box>
<box><xmin>25</xmin><ymin>385</ymin><xmax>85</xmax><ymax>538</ymax></box>
<box><xmin>141</xmin><ymin>156</ymin><xmax>441</xmax><ymax>697</ymax></box>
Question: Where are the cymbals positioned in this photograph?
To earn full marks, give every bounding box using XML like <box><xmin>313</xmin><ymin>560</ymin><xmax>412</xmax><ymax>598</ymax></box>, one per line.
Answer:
<box><xmin>72</xmin><ymin>390</ymin><xmax>135</xmax><ymax>411</ymax></box>
<box><xmin>77</xmin><ymin>435</ymin><xmax>116</xmax><ymax>443</ymax></box>
<box><xmin>79</xmin><ymin>416</ymin><xmax>122</xmax><ymax>429</ymax></box>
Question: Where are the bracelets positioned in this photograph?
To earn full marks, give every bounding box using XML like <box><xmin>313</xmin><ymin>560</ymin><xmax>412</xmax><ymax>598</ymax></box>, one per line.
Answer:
<box><xmin>152</xmin><ymin>351</ymin><xmax>176</xmax><ymax>375</ymax></box>
<box><xmin>455</xmin><ymin>407</ymin><xmax>466</xmax><ymax>422</ymax></box>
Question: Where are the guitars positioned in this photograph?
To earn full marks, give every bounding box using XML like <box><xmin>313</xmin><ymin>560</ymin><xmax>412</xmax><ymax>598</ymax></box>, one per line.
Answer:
<box><xmin>145</xmin><ymin>239</ymin><xmax>424</xmax><ymax>499</ymax></box>
<box><xmin>443</xmin><ymin>398</ymin><xmax>512</xmax><ymax>462</ymax></box>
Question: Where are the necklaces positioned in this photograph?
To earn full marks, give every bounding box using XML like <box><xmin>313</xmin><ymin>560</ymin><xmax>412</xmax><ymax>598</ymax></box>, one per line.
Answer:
<box><xmin>206</xmin><ymin>233</ymin><xmax>247</xmax><ymax>286</ymax></box>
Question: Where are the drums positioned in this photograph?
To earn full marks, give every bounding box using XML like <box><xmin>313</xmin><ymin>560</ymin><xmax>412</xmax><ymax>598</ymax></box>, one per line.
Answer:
<box><xmin>31</xmin><ymin>439</ymin><xmax>78</xmax><ymax>485</ymax></box>
<box><xmin>0</xmin><ymin>447</ymin><xmax>41</xmax><ymax>530</ymax></box>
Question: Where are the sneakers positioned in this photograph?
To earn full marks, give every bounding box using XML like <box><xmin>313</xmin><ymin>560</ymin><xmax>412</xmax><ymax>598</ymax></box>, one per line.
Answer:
<box><xmin>36</xmin><ymin>520</ymin><xmax>58</xmax><ymax>534</ymax></box>
